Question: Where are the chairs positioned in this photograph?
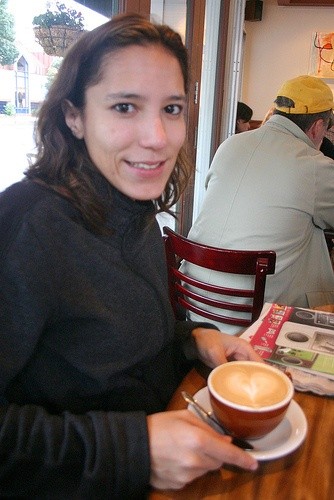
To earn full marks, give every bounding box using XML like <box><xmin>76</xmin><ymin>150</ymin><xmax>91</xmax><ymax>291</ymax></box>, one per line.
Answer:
<box><xmin>164</xmin><ymin>227</ymin><xmax>276</xmax><ymax>330</ymax></box>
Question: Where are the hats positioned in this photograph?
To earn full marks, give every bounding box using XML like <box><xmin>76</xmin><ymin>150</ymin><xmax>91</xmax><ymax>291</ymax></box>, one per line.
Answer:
<box><xmin>272</xmin><ymin>74</ymin><xmax>334</xmax><ymax>114</ymax></box>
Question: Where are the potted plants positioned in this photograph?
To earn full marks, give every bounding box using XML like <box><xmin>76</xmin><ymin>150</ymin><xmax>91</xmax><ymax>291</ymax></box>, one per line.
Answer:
<box><xmin>33</xmin><ymin>2</ymin><xmax>91</xmax><ymax>56</ymax></box>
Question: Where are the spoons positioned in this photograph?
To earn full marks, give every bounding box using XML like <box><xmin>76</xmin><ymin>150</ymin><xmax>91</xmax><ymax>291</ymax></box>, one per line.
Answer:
<box><xmin>181</xmin><ymin>390</ymin><xmax>254</xmax><ymax>451</ymax></box>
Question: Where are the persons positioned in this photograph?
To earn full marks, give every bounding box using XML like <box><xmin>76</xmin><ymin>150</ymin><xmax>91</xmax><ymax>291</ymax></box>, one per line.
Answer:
<box><xmin>180</xmin><ymin>75</ymin><xmax>334</xmax><ymax>334</ymax></box>
<box><xmin>236</xmin><ymin>102</ymin><xmax>253</xmax><ymax>132</ymax></box>
<box><xmin>0</xmin><ymin>14</ymin><xmax>264</xmax><ymax>500</ymax></box>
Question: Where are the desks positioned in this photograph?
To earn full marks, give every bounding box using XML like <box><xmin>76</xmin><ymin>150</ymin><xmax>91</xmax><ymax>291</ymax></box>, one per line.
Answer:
<box><xmin>151</xmin><ymin>303</ymin><xmax>334</xmax><ymax>500</ymax></box>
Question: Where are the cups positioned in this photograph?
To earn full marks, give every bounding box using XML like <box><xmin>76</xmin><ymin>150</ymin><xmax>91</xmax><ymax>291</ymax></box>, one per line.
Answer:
<box><xmin>206</xmin><ymin>361</ymin><xmax>295</xmax><ymax>441</ymax></box>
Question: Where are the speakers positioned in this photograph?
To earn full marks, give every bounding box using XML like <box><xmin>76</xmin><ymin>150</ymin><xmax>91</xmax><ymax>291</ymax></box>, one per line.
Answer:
<box><xmin>245</xmin><ymin>0</ymin><xmax>263</xmax><ymax>22</ymax></box>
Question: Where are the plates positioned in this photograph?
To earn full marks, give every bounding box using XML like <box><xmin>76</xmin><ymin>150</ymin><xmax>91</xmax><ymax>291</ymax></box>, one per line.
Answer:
<box><xmin>187</xmin><ymin>386</ymin><xmax>309</xmax><ymax>461</ymax></box>
